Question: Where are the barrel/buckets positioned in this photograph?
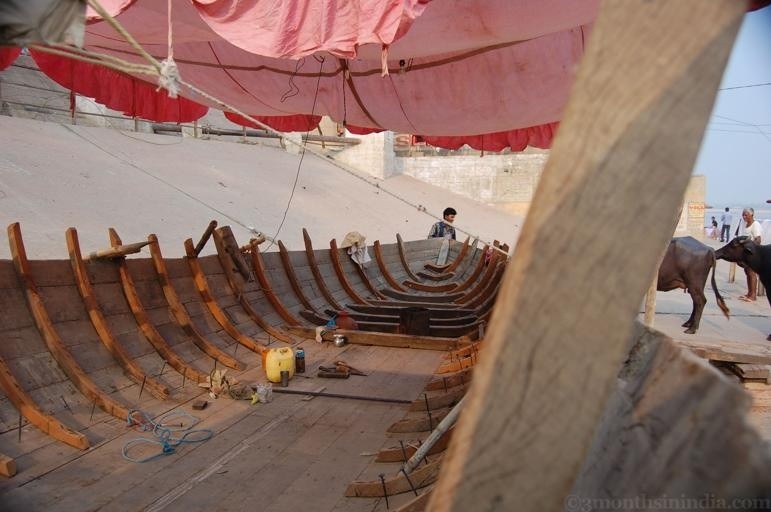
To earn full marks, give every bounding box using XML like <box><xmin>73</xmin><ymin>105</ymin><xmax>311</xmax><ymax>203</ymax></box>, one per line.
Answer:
<box><xmin>265</xmin><ymin>347</ymin><xmax>295</xmax><ymax>383</ymax></box>
<box><xmin>399</xmin><ymin>306</ymin><xmax>430</xmax><ymax>336</ymax></box>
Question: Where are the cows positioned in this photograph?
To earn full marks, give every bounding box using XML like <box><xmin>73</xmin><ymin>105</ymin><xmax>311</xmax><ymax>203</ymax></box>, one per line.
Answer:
<box><xmin>715</xmin><ymin>234</ymin><xmax>771</xmax><ymax>341</ymax></box>
<box><xmin>656</xmin><ymin>235</ymin><xmax>730</xmax><ymax>334</ymax></box>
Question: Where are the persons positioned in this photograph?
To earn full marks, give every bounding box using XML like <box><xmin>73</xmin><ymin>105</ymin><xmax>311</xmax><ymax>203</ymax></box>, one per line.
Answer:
<box><xmin>720</xmin><ymin>207</ymin><xmax>733</xmax><ymax>243</ymax></box>
<box><xmin>428</xmin><ymin>207</ymin><xmax>456</xmax><ymax>240</ymax></box>
<box><xmin>709</xmin><ymin>216</ymin><xmax>718</xmax><ymax>240</ymax></box>
<box><xmin>737</xmin><ymin>207</ymin><xmax>762</xmax><ymax>301</ymax></box>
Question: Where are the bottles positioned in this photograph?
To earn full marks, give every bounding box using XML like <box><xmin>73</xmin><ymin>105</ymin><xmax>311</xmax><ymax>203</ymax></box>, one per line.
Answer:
<box><xmin>294</xmin><ymin>346</ymin><xmax>306</xmax><ymax>373</ymax></box>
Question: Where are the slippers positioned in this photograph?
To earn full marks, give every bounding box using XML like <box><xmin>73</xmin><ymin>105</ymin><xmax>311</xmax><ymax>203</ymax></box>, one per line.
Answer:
<box><xmin>739</xmin><ymin>295</ymin><xmax>755</xmax><ymax>304</ymax></box>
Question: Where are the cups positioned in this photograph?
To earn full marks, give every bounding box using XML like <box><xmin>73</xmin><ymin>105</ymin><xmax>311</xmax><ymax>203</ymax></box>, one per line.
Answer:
<box><xmin>279</xmin><ymin>370</ymin><xmax>290</xmax><ymax>388</ymax></box>
